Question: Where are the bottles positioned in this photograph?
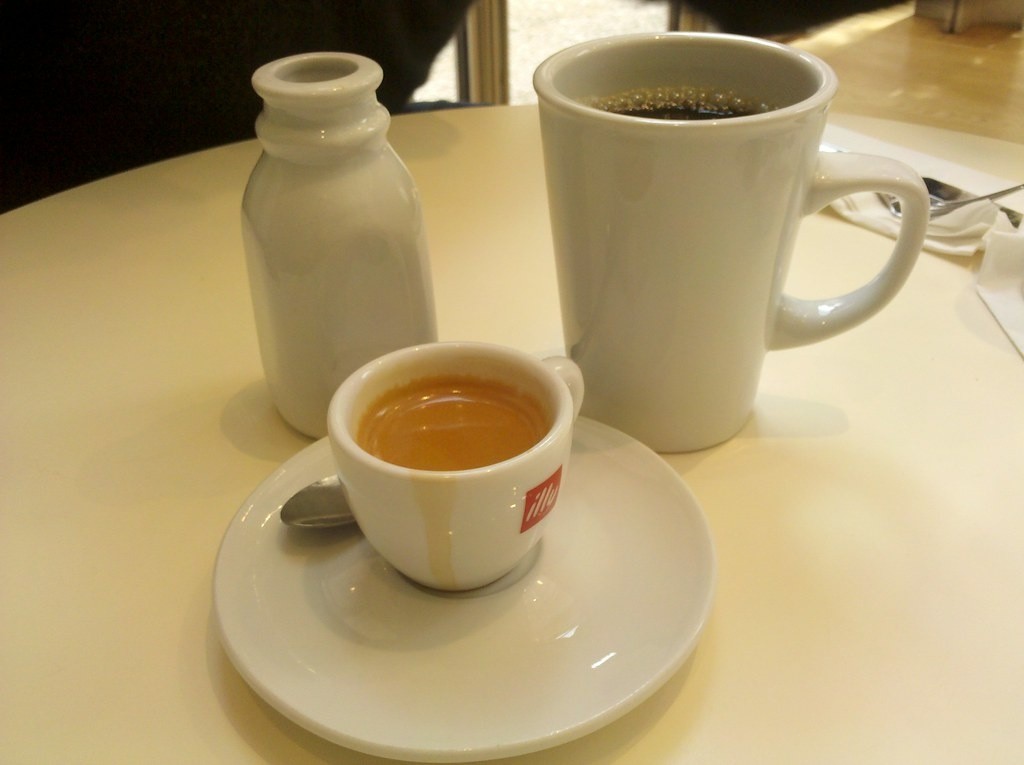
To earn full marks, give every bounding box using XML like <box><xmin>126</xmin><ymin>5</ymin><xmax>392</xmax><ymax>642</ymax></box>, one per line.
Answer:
<box><xmin>241</xmin><ymin>50</ymin><xmax>434</xmax><ymax>440</ymax></box>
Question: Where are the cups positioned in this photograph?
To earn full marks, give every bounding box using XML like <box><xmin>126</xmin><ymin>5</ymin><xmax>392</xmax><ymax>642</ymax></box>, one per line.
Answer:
<box><xmin>531</xmin><ymin>31</ymin><xmax>931</xmax><ymax>457</ymax></box>
<box><xmin>327</xmin><ymin>341</ymin><xmax>585</xmax><ymax>594</ymax></box>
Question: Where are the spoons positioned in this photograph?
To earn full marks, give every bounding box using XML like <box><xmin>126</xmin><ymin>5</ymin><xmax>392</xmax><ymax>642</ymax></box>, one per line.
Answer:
<box><xmin>279</xmin><ymin>474</ymin><xmax>359</xmax><ymax>529</ymax></box>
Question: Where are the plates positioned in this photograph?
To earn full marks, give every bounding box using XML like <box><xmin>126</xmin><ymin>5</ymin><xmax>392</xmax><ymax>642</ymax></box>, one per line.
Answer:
<box><xmin>213</xmin><ymin>412</ymin><xmax>716</xmax><ymax>764</ymax></box>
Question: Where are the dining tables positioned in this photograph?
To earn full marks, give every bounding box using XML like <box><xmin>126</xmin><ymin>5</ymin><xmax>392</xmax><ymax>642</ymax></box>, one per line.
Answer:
<box><xmin>0</xmin><ymin>103</ymin><xmax>1024</xmax><ymax>765</ymax></box>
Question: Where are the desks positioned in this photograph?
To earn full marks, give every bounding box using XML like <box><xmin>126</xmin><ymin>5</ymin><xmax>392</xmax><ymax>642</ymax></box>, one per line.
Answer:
<box><xmin>783</xmin><ymin>0</ymin><xmax>1024</xmax><ymax>214</ymax></box>
